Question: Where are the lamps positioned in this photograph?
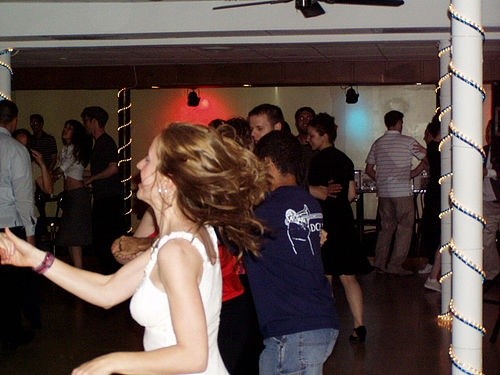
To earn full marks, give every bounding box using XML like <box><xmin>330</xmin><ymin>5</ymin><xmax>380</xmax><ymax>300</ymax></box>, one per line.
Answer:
<box><xmin>346</xmin><ymin>86</ymin><xmax>359</xmax><ymax>104</ymax></box>
<box><xmin>187</xmin><ymin>88</ymin><xmax>200</xmax><ymax>106</ymax></box>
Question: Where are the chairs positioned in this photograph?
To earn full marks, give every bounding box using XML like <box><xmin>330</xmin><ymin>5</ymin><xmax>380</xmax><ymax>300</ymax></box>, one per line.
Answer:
<box><xmin>37</xmin><ymin>187</ymin><xmax>93</xmax><ymax>256</ymax></box>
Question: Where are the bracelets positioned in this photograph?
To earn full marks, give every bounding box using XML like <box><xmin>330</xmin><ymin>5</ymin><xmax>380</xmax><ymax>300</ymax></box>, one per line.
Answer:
<box><xmin>34</xmin><ymin>251</ymin><xmax>55</xmax><ymax>274</ymax></box>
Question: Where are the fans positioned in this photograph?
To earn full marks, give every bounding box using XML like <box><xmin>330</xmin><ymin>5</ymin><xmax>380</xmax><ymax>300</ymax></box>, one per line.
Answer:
<box><xmin>213</xmin><ymin>0</ymin><xmax>405</xmax><ymax>18</ymax></box>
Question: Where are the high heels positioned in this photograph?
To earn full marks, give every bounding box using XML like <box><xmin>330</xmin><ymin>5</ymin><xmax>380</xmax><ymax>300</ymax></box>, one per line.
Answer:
<box><xmin>349</xmin><ymin>326</ymin><xmax>367</xmax><ymax>345</ymax></box>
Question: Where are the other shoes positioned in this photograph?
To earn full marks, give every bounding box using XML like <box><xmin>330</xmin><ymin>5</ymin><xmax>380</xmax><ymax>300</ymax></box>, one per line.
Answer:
<box><xmin>387</xmin><ymin>266</ymin><xmax>413</xmax><ymax>275</ymax></box>
<box><xmin>418</xmin><ymin>264</ymin><xmax>432</xmax><ymax>274</ymax></box>
<box><xmin>0</xmin><ymin>328</ymin><xmax>35</xmax><ymax>356</ymax></box>
<box><xmin>424</xmin><ymin>276</ymin><xmax>441</xmax><ymax>292</ymax></box>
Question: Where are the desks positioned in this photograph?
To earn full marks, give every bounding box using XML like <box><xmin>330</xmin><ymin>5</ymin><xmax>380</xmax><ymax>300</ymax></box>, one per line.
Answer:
<box><xmin>353</xmin><ymin>186</ymin><xmax>427</xmax><ymax>244</ymax></box>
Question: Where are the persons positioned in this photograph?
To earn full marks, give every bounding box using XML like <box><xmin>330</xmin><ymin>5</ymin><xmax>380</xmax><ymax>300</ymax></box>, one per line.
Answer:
<box><xmin>29</xmin><ymin>113</ymin><xmax>58</xmax><ymax>172</ymax></box>
<box><xmin>110</xmin><ymin>206</ymin><xmax>252</xmax><ymax>375</ymax></box>
<box><xmin>0</xmin><ymin>99</ymin><xmax>38</xmax><ymax>356</ymax></box>
<box><xmin>423</xmin><ymin>106</ymin><xmax>441</xmax><ymax>294</ymax></box>
<box><xmin>365</xmin><ymin>109</ymin><xmax>427</xmax><ymax>275</ymax></box>
<box><xmin>49</xmin><ymin>119</ymin><xmax>93</xmax><ymax>338</ymax></box>
<box><xmin>483</xmin><ymin>121</ymin><xmax>500</xmax><ymax>281</ymax></box>
<box><xmin>242</xmin><ymin>130</ymin><xmax>344</xmax><ymax>375</ymax></box>
<box><xmin>303</xmin><ymin>112</ymin><xmax>368</xmax><ymax>346</ymax></box>
<box><xmin>292</xmin><ymin>106</ymin><xmax>319</xmax><ymax>154</ymax></box>
<box><xmin>0</xmin><ymin>120</ymin><xmax>271</xmax><ymax>375</ymax></box>
<box><xmin>12</xmin><ymin>128</ymin><xmax>54</xmax><ymax>219</ymax></box>
<box><xmin>81</xmin><ymin>105</ymin><xmax>131</xmax><ymax>213</ymax></box>
<box><xmin>244</xmin><ymin>103</ymin><xmax>285</xmax><ymax>144</ymax></box>
